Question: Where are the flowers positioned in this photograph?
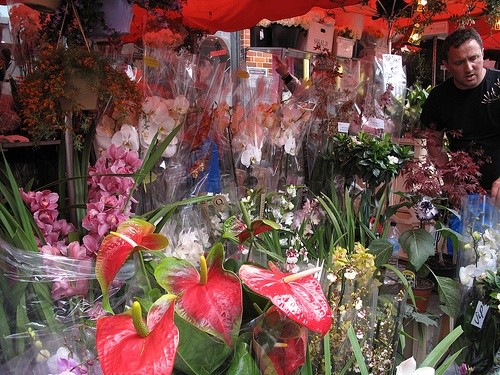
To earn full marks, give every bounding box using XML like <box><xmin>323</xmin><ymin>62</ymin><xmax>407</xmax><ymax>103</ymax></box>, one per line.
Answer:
<box><xmin>0</xmin><ymin>0</ymin><xmax>427</xmax><ymax>375</ymax></box>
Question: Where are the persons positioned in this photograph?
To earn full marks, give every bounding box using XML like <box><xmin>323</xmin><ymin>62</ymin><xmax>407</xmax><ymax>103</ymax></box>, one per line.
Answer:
<box><xmin>192</xmin><ymin>35</ymin><xmax>233</xmax><ymax>194</ymax></box>
<box><xmin>275</xmin><ymin>53</ymin><xmax>341</xmax><ymax>100</ymax></box>
<box><xmin>417</xmin><ymin>27</ymin><xmax>500</xmax><ymax>255</ymax></box>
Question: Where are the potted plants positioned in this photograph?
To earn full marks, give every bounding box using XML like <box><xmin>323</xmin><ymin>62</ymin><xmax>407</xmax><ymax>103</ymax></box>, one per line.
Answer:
<box><xmin>382</xmin><ymin>191</ymin><xmax>464</xmax><ymax>313</ymax></box>
<box><xmin>400</xmin><ymin>128</ymin><xmax>493</xmax><ymax>295</ymax></box>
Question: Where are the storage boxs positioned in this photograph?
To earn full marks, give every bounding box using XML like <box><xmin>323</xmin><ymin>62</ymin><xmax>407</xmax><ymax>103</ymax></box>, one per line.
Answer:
<box><xmin>296</xmin><ymin>22</ymin><xmax>333</xmax><ymax>54</ymax></box>
<box><xmin>332</xmin><ymin>36</ymin><xmax>356</xmax><ymax>59</ymax></box>
